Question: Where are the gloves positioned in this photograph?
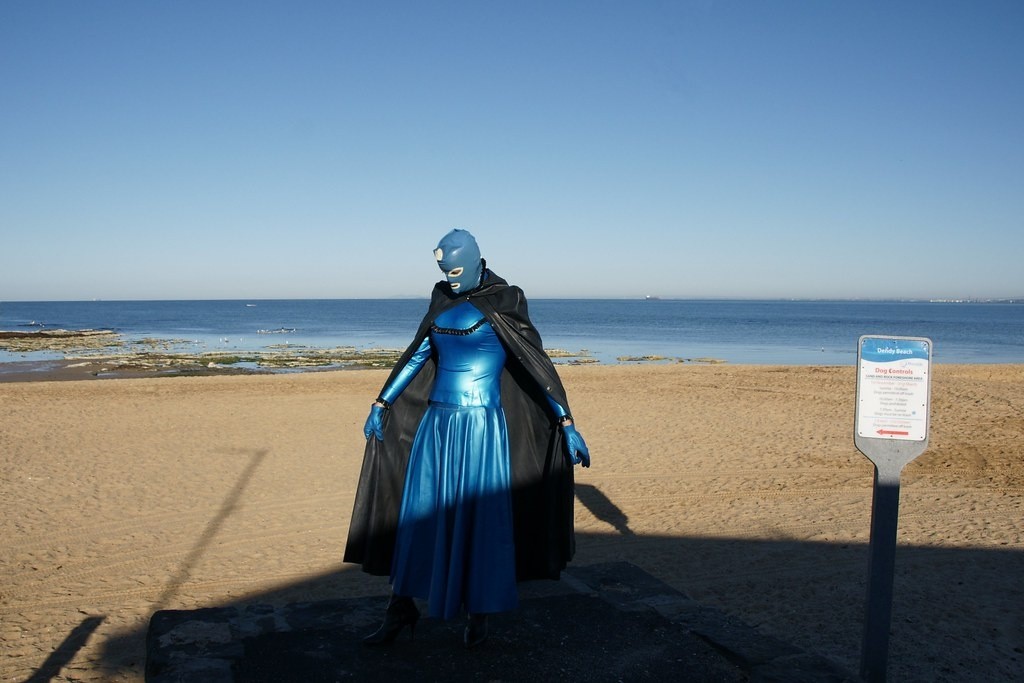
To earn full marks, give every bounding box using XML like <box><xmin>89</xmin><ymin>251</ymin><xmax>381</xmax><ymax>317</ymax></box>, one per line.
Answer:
<box><xmin>562</xmin><ymin>423</ymin><xmax>590</xmax><ymax>468</ymax></box>
<box><xmin>363</xmin><ymin>403</ymin><xmax>386</xmax><ymax>441</ymax></box>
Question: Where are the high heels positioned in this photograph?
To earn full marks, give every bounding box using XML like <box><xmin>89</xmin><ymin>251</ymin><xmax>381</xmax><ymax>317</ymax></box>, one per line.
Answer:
<box><xmin>362</xmin><ymin>594</ymin><xmax>422</xmax><ymax>647</ymax></box>
<box><xmin>464</xmin><ymin>614</ymin><xmax>489</xmax><ymax>649</ymax></box>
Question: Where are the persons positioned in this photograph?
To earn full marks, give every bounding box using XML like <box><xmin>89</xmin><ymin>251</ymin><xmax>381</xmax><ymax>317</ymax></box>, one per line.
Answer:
<box><xmin>343</xmin><ymin>230</ymin><xmax>590</xmax><ymax>644</ymax></box>
